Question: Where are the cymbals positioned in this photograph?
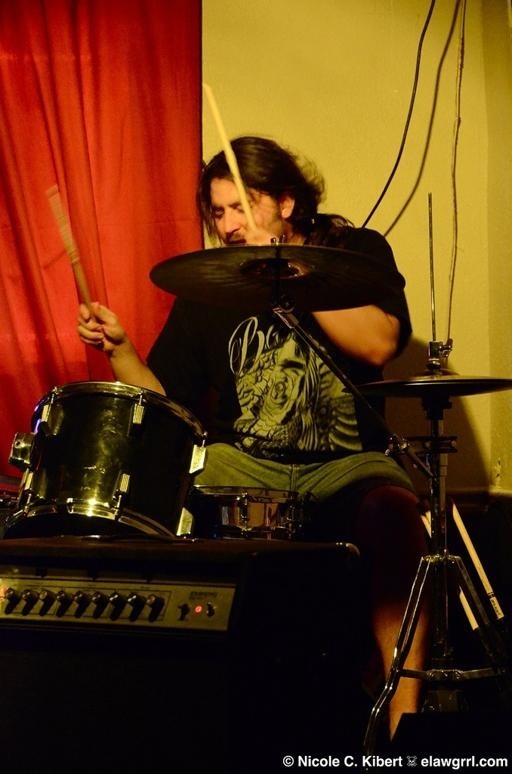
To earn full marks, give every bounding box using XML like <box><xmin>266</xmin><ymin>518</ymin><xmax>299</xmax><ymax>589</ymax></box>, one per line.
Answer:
<box><xmin>149</xmin><ymin>245</ymin><xmax>374</xmax><ymax>307</ymax></box>
<box><xmin>341</xmin><ymin>375</ymin><xmax>512</xmax><ymax>398</ymax></box>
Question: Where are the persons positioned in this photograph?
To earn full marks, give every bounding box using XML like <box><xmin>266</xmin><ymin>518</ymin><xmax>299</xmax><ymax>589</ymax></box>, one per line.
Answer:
<box><xmin>76</xmin><ymin>136</ymin><xmax>430</xmax><ymax>768</ymax></box>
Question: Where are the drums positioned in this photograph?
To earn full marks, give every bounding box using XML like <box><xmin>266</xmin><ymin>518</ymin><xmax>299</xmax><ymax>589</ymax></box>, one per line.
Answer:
<box><xmin>0</xmin><ymin>382</ymin><xmax>207</xmax><ymax>540</ymax></box>
<box><xmin>191</xmin><ymin>483</ymin><xmax>301</xmax><ymax>540</ymax></box>
